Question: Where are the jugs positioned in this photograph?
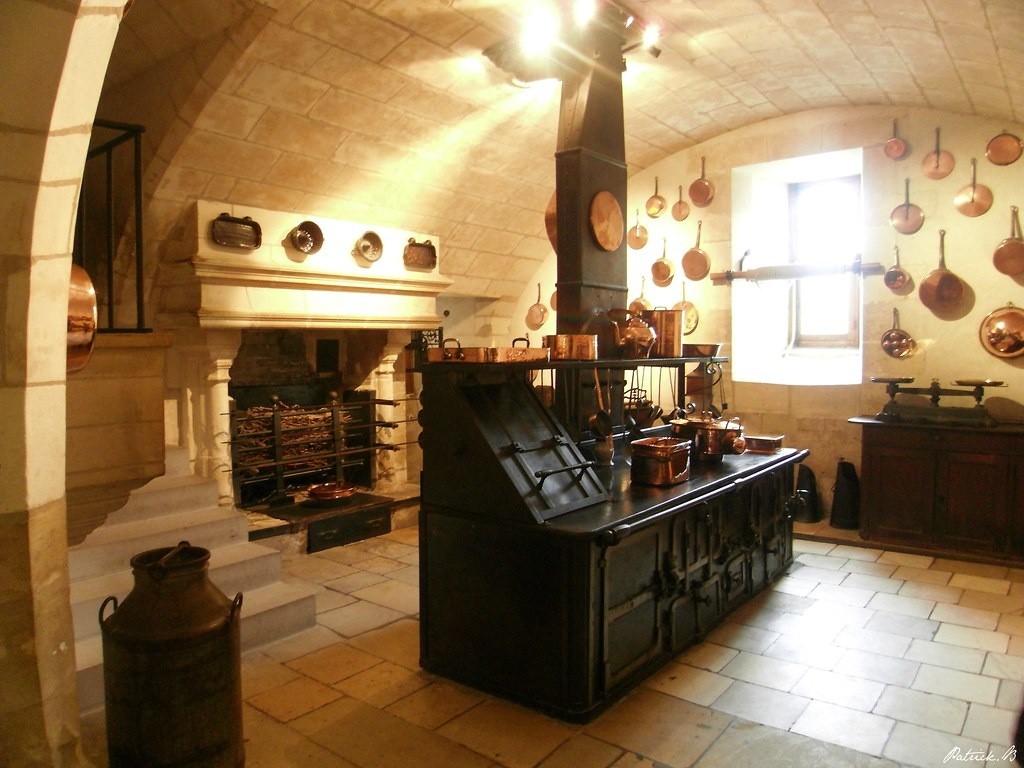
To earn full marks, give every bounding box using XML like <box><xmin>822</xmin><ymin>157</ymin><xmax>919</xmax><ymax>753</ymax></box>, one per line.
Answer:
<box><xmin>607</xmin><ymin>309</ymin><xmax>657</xmax><ymax>359</ymax></box>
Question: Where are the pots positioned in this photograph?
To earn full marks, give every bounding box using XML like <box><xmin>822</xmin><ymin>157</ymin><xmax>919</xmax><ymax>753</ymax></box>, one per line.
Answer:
<box><xmin>671</xmin><ymin>412</ymin><xmax>745</xmax><ymax>464</ymax></box>
<box><xmin>624</xmin><ymin>400</ymin><xmax>663</xmax><ymax>431</ymax></box>
<box><xmin>627</xmin><ymin>155</ymin><xmax>714</xmax><ymax>334</ymax></box>
<box><xmin>307</xmin><ymin>482</ymin><xmax>360</xmax><ymax>507</ymax></box>
<box><xmin>528</xmin><ymin>283</ymin><xmax>549</xmax><ymax>325</ymax></box>
<box><xmin>589</xmin><ymin>367</ymin><xmax>613</xmax><ymax>439</ymax></box>
<box><xmin>880</xmin><ymin>117</ymin><xmax>1024</xmax><ymax>359</ymax></box>
<box><xmin>629</xmin><ymin>437</ymin><xmax>693</xmax><ymax>486</ymax></box>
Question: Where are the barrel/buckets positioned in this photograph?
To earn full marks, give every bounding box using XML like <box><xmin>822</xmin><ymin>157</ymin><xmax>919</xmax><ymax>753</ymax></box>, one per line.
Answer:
<box><xmin>641</xmin><ymin>307</ymin><xmax>683</xmax><ymax>357</ymax></box>
<box><xmin>98</xmin><ymin>548</ymin><xmax>245</xmax><ymax>768</ymax></box>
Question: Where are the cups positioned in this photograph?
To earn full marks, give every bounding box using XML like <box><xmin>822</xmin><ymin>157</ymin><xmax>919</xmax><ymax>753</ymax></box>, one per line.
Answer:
<box><xmin>571</xmin><ymin>334</ymin><xmax>598</xmax><ymax>360</ymax></box>
<box><xmin>543</xmin><ymin>334</ymin><xmax>571</xmax><ymax>360</ymax></box>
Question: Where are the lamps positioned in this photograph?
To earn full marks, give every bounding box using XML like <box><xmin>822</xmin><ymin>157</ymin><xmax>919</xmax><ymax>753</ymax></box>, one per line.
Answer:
<box><xmin>621</xmin><ymin>41</ymin><xmax>661</xmax><ymax>58</ymax></box>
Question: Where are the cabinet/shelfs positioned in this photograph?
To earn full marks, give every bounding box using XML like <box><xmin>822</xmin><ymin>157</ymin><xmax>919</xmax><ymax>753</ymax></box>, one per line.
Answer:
<box><xmin>420</xmin><ymin>357</ymin><xmax>810</xmax><ymax>725</ymax></box>
<box><xmin>847</xmin><ymin>415</ymin><xmax>1024</xmax><ymax>566</ymax></box>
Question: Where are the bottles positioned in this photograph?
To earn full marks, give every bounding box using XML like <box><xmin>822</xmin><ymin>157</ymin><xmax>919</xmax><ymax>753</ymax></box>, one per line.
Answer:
<box><xmin>593</xmin><ymin>436</ymin><xmax>612</xmax><ymax>467</ymax></box>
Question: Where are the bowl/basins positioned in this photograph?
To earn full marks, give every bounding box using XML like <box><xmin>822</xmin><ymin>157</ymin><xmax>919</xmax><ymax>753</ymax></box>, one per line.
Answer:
<box><xmin>683</xmin><ymin>343</ymin><xmax>719</xmax><ymax>357</ymax></box>
<box><xmin>744</xmin><ymin>436</ymin><xmax>784</xmax><ymax>453</ymax></box>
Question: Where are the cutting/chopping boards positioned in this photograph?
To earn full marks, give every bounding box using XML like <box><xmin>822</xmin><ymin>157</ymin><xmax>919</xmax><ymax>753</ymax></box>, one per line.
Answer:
<box><xmin>590</xmin><ymin>190</ymin><xmax>624</xmax><ymax>252</ymax></box>
